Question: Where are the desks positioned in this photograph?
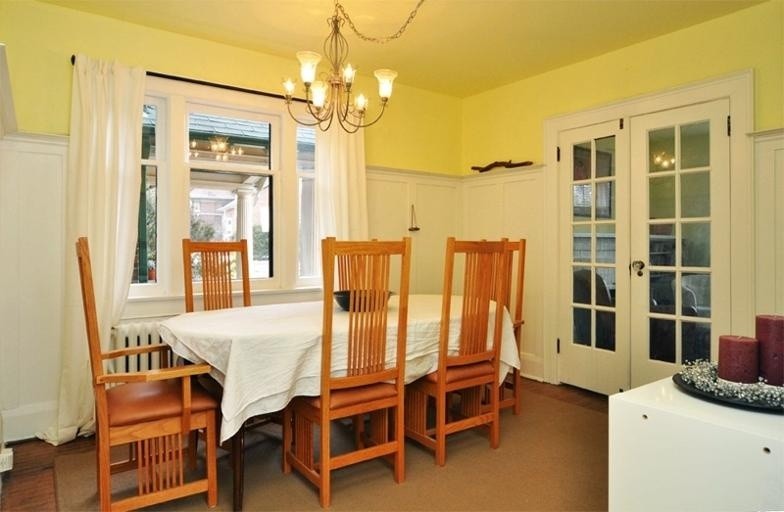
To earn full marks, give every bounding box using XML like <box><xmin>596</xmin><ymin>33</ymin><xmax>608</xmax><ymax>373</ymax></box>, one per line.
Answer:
<box><xmin>606</xmin><ymin>375</ymin><xmax>783</xmax><ymax>511</ymax></box>
<box><xmin>158</xmin><ymin>294</ymin><xmax>507</xmax><ymax>512</ymax></box>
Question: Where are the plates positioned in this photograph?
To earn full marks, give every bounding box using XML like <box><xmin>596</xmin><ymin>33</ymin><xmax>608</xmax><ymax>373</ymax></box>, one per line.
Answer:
<box><xmin>672</xmin><ymin>372</ymin><xmax>783</xmax><ymax>415</ymax></box>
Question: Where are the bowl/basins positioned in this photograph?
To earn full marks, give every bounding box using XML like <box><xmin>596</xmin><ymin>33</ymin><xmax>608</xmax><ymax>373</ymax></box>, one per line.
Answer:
<box><xmin>333</xmin><ymin>289</ymin><xmax>393</xmax><ymax>312</ymax></box>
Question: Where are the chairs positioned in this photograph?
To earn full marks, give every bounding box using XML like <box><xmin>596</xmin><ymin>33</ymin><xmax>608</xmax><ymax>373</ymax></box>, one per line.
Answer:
<box><xmin>404</xmin><ymin>238</ymin><xmax>509</xmax><ymax>467</ymax></box>
<box><xmin>482</xmin><ymin>239</ymin><xmax>525</xmax><ymax>415</ymax></box>
<box><xmin>76</xmin><ymin>236</ymin><xmax>220</xmax><ymax>512</ymax></box>
<box><xmin>283</xmin><ymin>237</ymin><xmax>412</xmax><ymax>509</ymax></box>
<box><xmin>182</xmin><ymin>238</ymin><xmax>283</xmax><ymax>467</ymax></box>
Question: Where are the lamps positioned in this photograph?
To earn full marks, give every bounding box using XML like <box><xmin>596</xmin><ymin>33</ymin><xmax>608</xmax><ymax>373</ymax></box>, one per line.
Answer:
<box><xmin>282</xmin><ymin>1</ymin><xmax>399</xmax><ymax>134</ymax></box>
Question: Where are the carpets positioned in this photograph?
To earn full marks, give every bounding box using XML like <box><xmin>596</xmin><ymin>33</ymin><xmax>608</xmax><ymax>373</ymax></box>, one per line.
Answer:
<box><xmin>53</xmin><ymin>385</ymin><xmax>608</xmax><ymax>511</ymax></box>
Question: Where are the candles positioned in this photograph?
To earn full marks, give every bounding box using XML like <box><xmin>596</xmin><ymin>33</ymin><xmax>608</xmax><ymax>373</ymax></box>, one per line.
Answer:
<box><xmin>719</xmin><ymin>313</ymin><xmax>784</xmax><ymax>385</ymax></box>
